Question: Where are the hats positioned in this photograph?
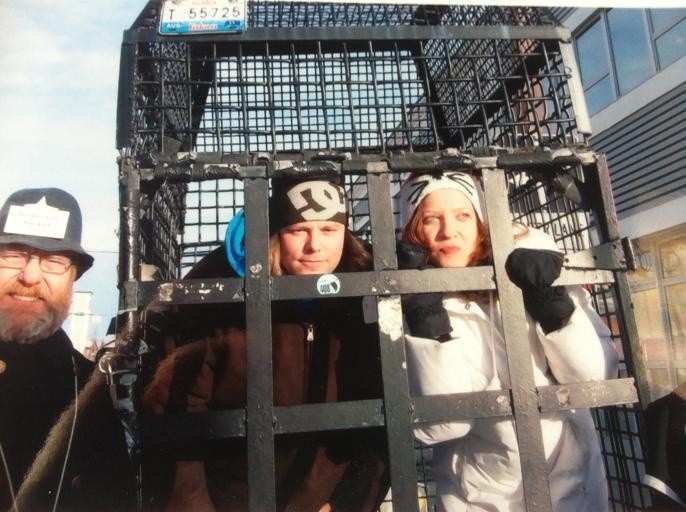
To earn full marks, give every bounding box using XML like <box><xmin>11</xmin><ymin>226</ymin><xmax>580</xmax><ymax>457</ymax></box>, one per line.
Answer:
<box><xmin>272</xmin><ymin>176</ymin><xmax>349</xmax><ymax>228</ymax></box>
<box><xmin>400</xmin><ymin>163</ymin><xmax>488</xmax><ymax>236</ymax></box>
<box><xmin>0</xmin><ymin>187</ymin><xmax>94</xmax><ymax>281</ymax></box>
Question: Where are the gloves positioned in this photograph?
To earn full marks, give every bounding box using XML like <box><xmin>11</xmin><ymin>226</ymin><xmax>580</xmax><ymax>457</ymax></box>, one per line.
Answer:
<box><xmin>504</xmin><ymin>247</ymin><xmax>574</xmax><ymax>334</ymax></box>
<box><xmin>395</xmin><ymin>240</ymin><xmax>452</xmax><ymax>342</ymax></box>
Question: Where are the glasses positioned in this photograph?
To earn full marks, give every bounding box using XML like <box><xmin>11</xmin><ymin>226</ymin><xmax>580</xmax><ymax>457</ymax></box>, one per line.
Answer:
<box><xmin>2</xmin><ymin>252</ymin><xmax>77</xmax><ymax>276</ymax></box>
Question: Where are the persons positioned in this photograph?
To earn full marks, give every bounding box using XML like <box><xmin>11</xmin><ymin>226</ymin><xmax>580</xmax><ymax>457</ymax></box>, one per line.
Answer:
<box><xmin>362</xmin><ymin>169</ymin><xmax>622</xmax><ymax>511</ymax></box>
<box><xmin>1</xmin><ymin>182</ymin><xmax>135</xmax><ymax>510</ymax></box>
<box><xmin>139</xmin><ymin>175</ymin><xmax>411</xmax><ymax>511</ymax></box>
